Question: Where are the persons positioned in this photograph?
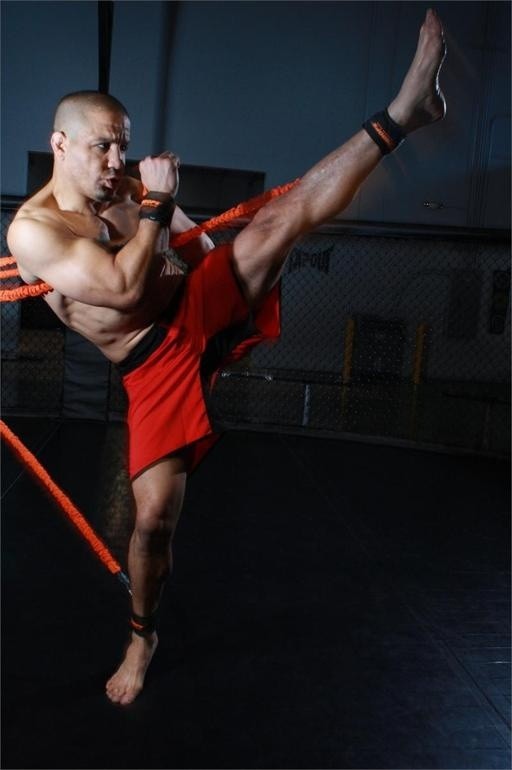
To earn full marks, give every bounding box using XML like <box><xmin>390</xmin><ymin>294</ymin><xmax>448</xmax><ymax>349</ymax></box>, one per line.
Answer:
<box><xmin>6</xmin><ymin>4</ymin><xmax>450</xmax><ymax>712</ymax></box>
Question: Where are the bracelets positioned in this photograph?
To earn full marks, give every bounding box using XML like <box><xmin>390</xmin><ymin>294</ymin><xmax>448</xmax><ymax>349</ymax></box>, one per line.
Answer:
<box><xmin>135</xmin><ymin>191</ymin><xmax>176</xmax><ymax>230</ymax></box>
